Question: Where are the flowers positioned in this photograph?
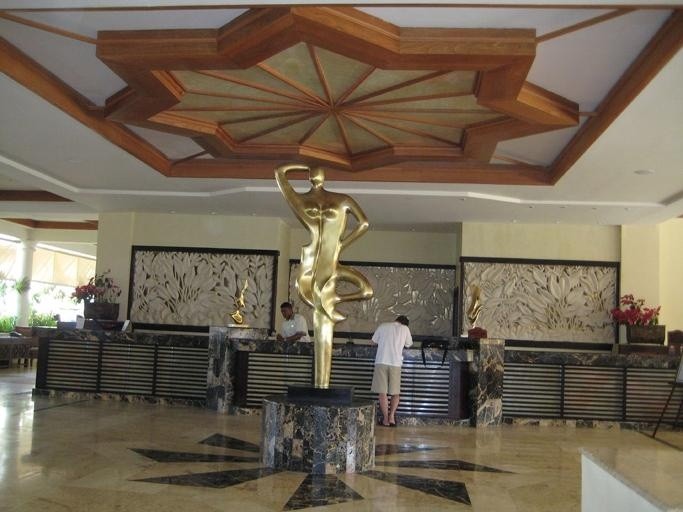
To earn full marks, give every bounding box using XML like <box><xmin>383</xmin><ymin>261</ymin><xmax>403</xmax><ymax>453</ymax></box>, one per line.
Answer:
<box><xmin>611</xmin><ymin>294</ymin><xmax>661</xmax><ymax>331</ymax></box>
<box><xmin>70</xmin><ymin>269</ymin><xmax>122</xmax><ymax>303</ymax></box>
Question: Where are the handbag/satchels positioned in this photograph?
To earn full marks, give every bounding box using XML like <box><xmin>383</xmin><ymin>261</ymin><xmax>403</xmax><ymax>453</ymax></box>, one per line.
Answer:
<box><xmin>423</xmin><ymin>337</ymin><xmax>448</xmax><ymax>349</ymax></box>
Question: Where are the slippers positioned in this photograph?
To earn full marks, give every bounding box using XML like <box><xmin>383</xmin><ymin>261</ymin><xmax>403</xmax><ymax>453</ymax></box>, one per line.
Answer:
<box><xmin>378</xmin><ymin>418</ymin><xmax>397</xmax><ymax>427</ymax></box>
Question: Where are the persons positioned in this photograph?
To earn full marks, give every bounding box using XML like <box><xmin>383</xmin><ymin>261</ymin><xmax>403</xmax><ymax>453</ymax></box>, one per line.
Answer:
<box><xmin>370</xmin><ymin>316</ymin><xmax>414</xmax><ymax>427</ymax></box>
<box><xmin>276</xmin><ymin>302</ymin><xmax>310</xmax><ymax>344</ymax></box>
<box><xmin>368</xmin><ymin>425</ymin><xmax>403</xmax><ymax>512</ymax></box>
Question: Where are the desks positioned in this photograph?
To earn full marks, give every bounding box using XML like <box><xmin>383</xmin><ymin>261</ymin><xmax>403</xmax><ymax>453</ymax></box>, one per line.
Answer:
<box><xmin>0</xmin><ymin>336</ymin><xmax>32</xmax><ymax>368</ymax></box>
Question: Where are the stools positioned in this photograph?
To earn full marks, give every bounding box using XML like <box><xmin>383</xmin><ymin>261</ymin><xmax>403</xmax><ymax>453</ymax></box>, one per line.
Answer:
<box><xmin>29</xmin><ymin>347</ymin><xmax>39</xmax><ymax>366</ymax></box>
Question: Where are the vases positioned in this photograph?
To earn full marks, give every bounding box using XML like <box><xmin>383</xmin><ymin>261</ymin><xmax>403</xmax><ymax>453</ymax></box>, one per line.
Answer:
<box><xmin>16</xmin><ymin>325</ymin><xmax>32</xmax><ymax>336</ymax></box>
<box><xmin>84</xmin><ymin>303</ymin><xmax>119</xmax><ymax>320</ymax></box>
<box><xmin>626</xmin><ymin>325</ymin><xmax>666</xmax><ymax>347</ymax></box>
<box><xmin>34</xmin><ymin>326</ymin><xmax>57</xmax><ymax>338</ymax></box>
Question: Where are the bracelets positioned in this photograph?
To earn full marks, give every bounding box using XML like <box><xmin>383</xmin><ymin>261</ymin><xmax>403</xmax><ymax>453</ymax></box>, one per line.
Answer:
<box><xmin>284</xmin><ymin>338</ymin><xmax>288</xmax><ymax>342</ymax></box>
